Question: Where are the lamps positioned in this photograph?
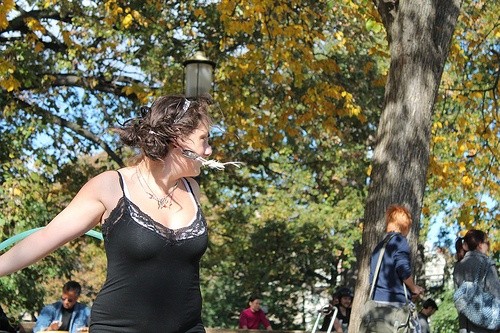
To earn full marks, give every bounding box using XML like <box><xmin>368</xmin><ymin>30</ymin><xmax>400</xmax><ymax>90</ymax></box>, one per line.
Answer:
<box><xmin>182</xmin><ymin>49</ymin><xmax>217</xmax><ymax>104</ymax></box>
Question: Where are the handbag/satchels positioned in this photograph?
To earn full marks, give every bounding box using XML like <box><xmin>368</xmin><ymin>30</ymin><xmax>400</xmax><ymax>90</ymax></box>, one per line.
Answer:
<box><xmin>453</xmin><ymin>259</ymin><xmax>500</xmax><ymax>330</ymax></box>
<box><xmin>358</xmin><ymin>299</ymin><xmax>412</xmax><ymax>333</ymax></box>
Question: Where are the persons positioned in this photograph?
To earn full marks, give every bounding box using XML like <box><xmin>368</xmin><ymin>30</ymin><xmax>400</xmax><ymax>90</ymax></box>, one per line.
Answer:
<box><xmin>453</xmin><ymin>229</ymin><xmax>500</xmax><ymax>333</ymax></box>
<box><xmin>238</xmin><ymin>293</ymin><xmax>272</xmax><ymax>333</ymax></box>
<box><xmin>418</xmin><ymin>298</ymin><xmax>438</xmax><ymax>333</ymax></box>
<box><xmin>0</xmin><ymin>95</ymin><xmax>214</xmax><ymax>333</ymax></box>
<box><xmin>358</xmin><ymin>205</ymin><xmax>424</xmax><ymax>333</ymax></box>
<box><xmin>323</xmin><ymin>287</ymin><xmax>353</xmax><ymax>333</ymax></box>
<box><xmin>32</xmin><ymin>281</ymin><xmax>90</xmax><ymax>333</ymax></box>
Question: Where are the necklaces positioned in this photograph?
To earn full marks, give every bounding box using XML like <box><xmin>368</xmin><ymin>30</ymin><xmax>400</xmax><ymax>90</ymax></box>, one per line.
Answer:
<box><xmin>135</xmin><ymin>166</ymin><xmax>178</xmax><ymax>207</ymax></box>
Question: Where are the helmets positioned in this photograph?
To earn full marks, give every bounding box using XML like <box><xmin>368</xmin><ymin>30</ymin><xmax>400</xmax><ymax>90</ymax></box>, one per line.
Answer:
<box><xmin>337</xmin><ymin>287</ymin><xmax>353</xmax><ymax>297</ymax></box>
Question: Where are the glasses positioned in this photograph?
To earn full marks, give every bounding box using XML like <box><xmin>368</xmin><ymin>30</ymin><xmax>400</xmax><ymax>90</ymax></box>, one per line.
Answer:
<box><xmin>481</xmin><ymin>241</ymin><xmax>489</xmax><ymax>247</ymax></box>
<box><xmin>61</xmin><ymin>295</ymin><xmax>78</xmax><ymax>302</ymax></box>
